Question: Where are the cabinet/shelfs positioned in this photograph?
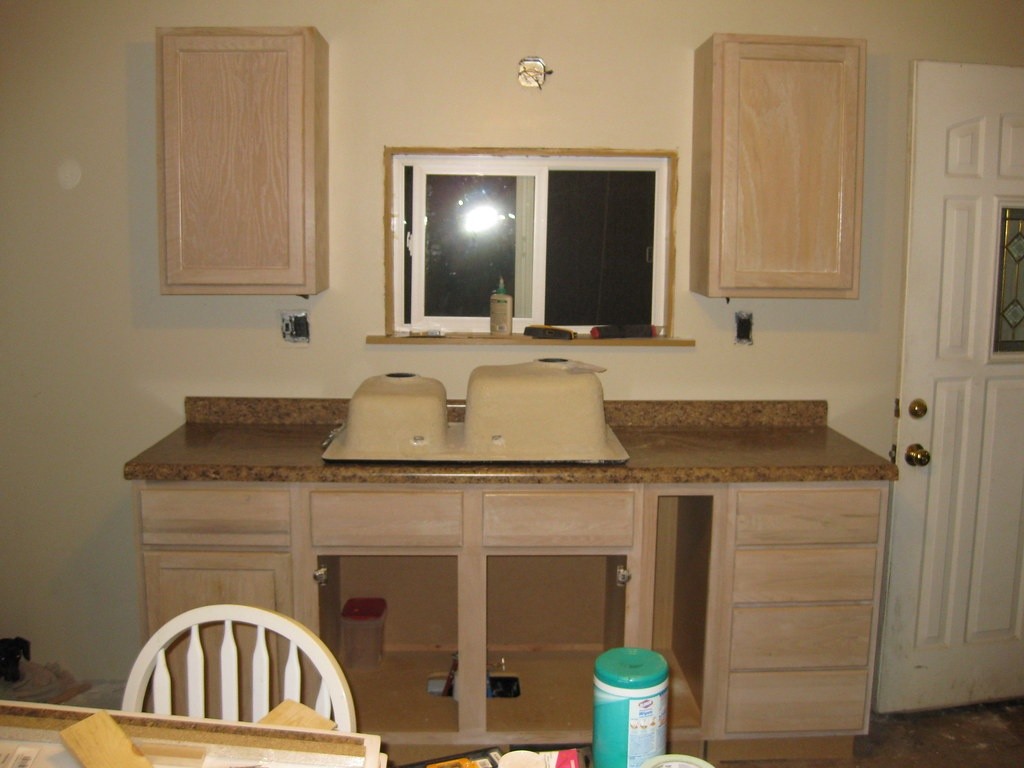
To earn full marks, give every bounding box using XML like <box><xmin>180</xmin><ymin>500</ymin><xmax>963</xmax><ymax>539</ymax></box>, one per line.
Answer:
<box><xmin>157</xmin><ymin>23</ymin><xmax>336</xmax><ymax>298</ymax></box>
<box><xmin>308</xmin><ymin>484</ymin><xmax>646</xmax><ymax>745</ymax></box>
<box><xmin>643</xmin><ymin>483</ymin><xmax>726</xmax><ymax>741</ymax></box>
<box><xmin>144</xmin><ymin>498</ymin><xmax>307</xmax><ymax>718</ymax></box>
<box><xmin>689</xmin><ymin>32</ymin><xmax>869</xmax><ymax>297</ymax></box>
<box><xmin>712</xmin><ymin>482</ymin><xmax>888</xmax><ymax>739</ymax></box>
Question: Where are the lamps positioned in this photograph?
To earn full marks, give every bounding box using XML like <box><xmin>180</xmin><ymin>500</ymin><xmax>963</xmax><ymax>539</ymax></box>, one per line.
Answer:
<box><xmin>517</xmin><ymin>56</ymin><xmax>554</xmax><ymax>91</ymax></box>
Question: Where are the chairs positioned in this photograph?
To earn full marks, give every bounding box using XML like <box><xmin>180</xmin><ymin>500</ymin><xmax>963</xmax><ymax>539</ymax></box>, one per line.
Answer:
<box><xmin>122</xmin><ymin>604</ymin><xmax>360</xmax><ymax>730</ymax></box>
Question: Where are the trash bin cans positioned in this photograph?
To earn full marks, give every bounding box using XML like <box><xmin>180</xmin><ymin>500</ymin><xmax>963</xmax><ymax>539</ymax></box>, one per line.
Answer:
<box><xmin>345</xmin><ymin>598</ymin><xmax>387</xmax><ymax>659</ymax></box>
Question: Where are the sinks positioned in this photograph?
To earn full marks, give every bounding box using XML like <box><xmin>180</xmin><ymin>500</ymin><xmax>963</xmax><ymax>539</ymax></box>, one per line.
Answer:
<box><xmin>342</xmin><ymin>371</ymin><xmax>451</xmax><ymax>456</ymax></box>
<box><xmin>462</xmin><ymin>357</ymin><xmax>609</xmax><ymax>458</ymax></box>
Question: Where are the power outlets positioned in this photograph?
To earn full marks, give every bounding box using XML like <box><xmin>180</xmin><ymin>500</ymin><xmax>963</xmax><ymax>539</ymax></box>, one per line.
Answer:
<box><xmin>281</xmin><ymin>309</ymin><xmax>311</xmax><ymax>343</ymax></box>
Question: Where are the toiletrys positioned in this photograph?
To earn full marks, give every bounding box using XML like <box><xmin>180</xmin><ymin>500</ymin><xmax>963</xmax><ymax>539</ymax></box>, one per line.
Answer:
<box><xmin>489</xmin><ymin>273</ymin><xmax>514</xmax><ymax>336</ymax></box>
